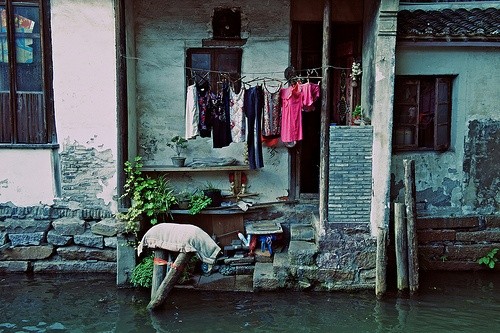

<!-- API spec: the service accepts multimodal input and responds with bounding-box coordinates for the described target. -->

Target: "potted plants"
[166,136,189,168]
[352,105,362,125]
[117,156,222,287]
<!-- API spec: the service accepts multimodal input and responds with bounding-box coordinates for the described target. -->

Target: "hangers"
[185,69,323,83]
